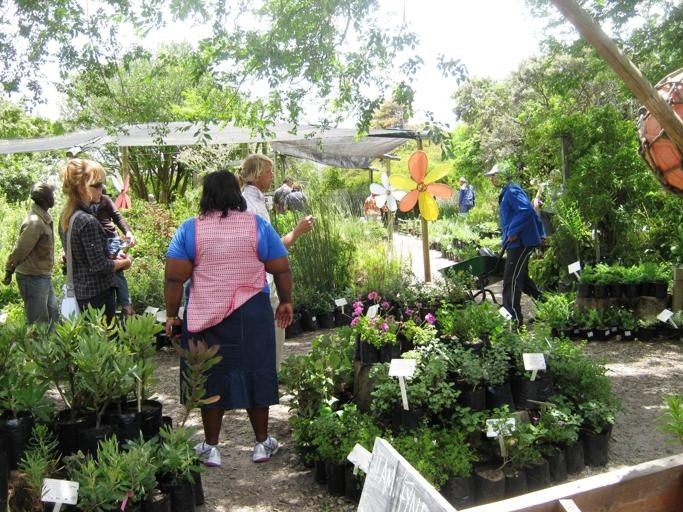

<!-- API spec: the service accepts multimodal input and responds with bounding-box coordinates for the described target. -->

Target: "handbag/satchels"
[61,285,80,317]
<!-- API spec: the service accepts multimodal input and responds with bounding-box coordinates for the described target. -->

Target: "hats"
[484,164,498,176]
[32,183,56,202]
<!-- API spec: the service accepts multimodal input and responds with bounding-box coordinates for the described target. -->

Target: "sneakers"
[253,437,277,460]
[192,442,220,466]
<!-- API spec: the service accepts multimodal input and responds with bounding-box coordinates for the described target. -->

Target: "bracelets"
[166,316,179,320]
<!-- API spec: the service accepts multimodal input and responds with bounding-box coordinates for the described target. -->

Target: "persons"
[234,155,315,373]
[88,196,136,314]
[273,176,294,211]
[459,177,475,213]
[486,165,549,327]
[283,183,309,212]
[534,169,564,238]
[59,159,131,325]
[164,169,293,468]
[363,193,388,218]
[2,186,58,325]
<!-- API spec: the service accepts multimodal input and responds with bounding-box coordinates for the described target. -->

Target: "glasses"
[88,182,102,190]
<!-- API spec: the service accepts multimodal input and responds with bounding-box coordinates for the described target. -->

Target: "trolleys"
[438,236,510,309]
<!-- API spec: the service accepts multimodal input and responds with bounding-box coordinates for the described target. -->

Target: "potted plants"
[277,261,683,511]
[0,300,224,511]
